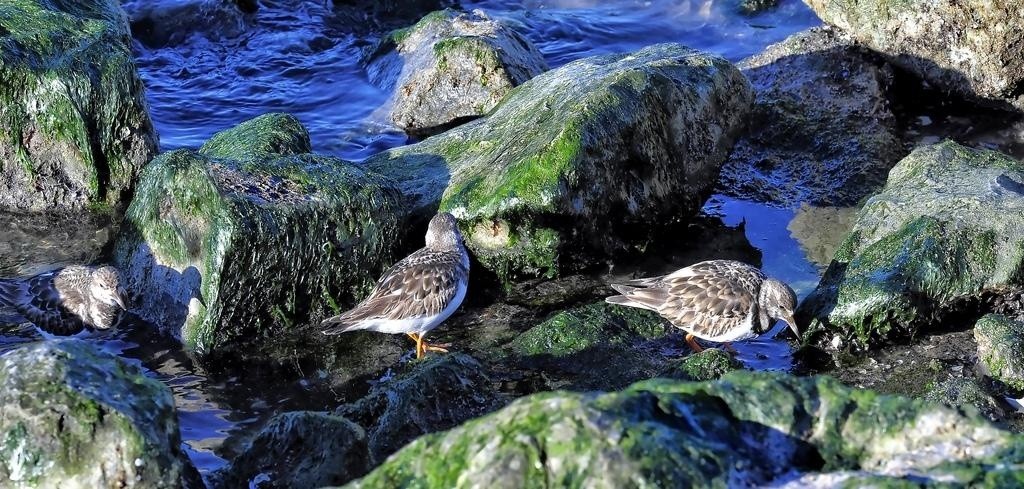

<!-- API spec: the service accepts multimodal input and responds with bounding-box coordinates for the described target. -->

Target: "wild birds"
[603,258,803,356]
[0,263,130,341]
[317,212,472,360]
